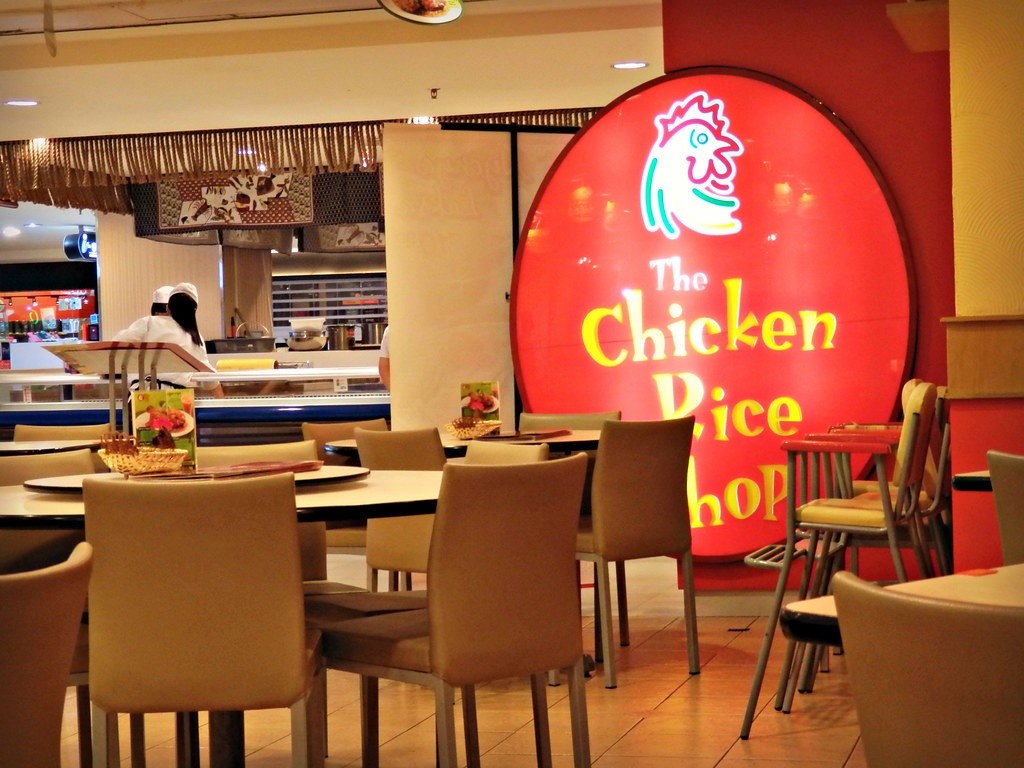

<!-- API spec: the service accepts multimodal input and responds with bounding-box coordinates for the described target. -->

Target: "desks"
[0,439,127,454]
[1,470,441,768]
[325,428,600,457]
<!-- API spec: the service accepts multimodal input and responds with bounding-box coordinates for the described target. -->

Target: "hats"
[152,286,174,303]
[168,282,199,310]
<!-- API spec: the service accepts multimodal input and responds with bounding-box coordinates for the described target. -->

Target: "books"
[127,459,326,481]
[476,430,573,441]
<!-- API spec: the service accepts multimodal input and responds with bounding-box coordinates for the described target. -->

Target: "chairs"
[0,380,1024,768]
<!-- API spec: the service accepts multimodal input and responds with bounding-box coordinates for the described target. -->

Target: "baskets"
[96,432,189,475]
[445,417,502,439]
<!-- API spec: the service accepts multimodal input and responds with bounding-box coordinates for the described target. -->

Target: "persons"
[378,325,389,392]
[112,282,224,443]
[256,380,287,396]
